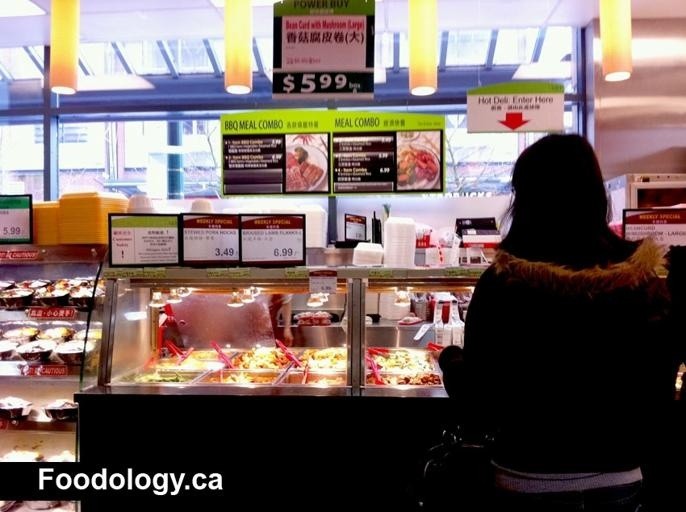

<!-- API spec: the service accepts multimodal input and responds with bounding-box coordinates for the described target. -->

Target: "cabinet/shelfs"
[0,243,151,512]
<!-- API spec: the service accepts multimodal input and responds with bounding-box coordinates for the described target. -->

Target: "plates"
[286,144,330,191]
[398,144,441,191]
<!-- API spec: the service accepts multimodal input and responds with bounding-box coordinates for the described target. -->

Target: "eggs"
[295,311,332,325]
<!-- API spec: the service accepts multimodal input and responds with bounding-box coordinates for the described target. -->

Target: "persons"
[432,131,685,512]
[266,291,297,347]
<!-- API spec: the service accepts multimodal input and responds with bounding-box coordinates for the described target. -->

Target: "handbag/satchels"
[419,443,493,512]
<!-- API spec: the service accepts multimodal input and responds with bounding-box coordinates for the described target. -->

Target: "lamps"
[595,0,634,84]
[143,284,413,317]
[403,0,441,98]
[46,0,82,96]
[222,0,254,98]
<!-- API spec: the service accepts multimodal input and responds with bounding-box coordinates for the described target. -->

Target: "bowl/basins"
[0,275,104,425]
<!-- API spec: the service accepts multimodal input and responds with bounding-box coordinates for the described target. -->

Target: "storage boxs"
[25,197,61,245]
[59,190,126,244]
